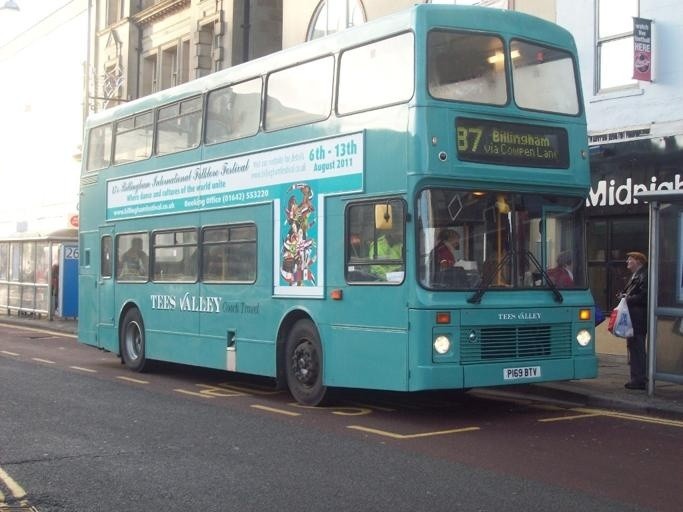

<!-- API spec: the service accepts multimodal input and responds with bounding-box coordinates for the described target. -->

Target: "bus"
[76,3,598,408]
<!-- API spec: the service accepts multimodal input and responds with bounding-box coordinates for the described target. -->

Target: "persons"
[615,250,649,391]
[427,230,460,285]
[369,228,403,284]
[545,251,574,287]
[122,238,149,277]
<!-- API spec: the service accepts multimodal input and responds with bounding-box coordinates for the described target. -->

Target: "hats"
[625,251,647,265]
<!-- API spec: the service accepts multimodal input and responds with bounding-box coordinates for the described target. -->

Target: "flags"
[630,16,652,82]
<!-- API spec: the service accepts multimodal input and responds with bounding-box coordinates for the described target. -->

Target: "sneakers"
[624,381,646,390]
